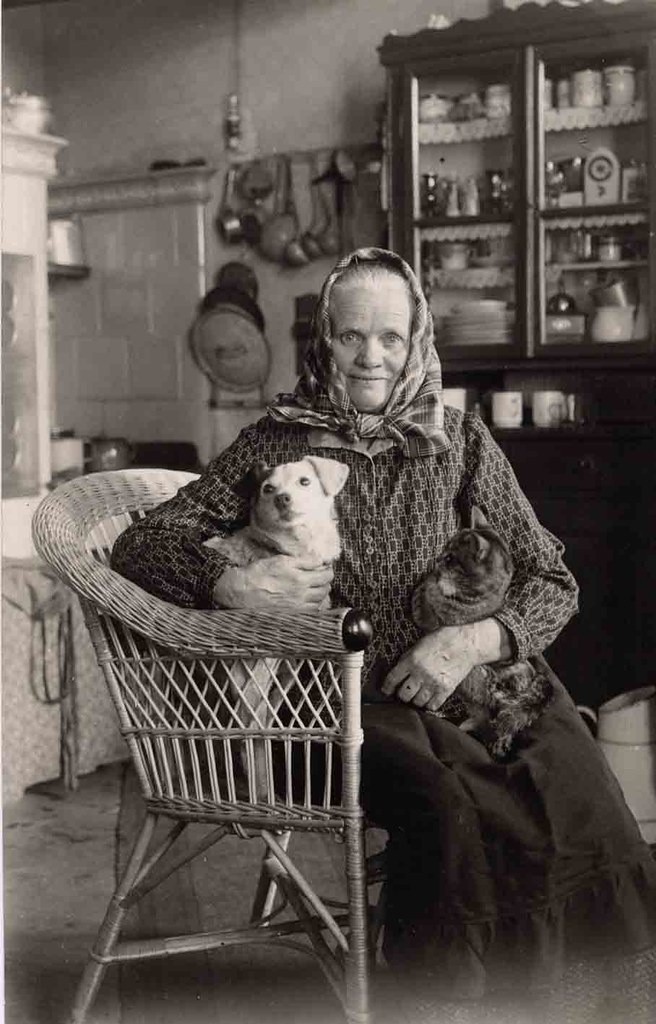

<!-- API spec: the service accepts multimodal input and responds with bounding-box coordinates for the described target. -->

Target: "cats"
[408,505,555,763]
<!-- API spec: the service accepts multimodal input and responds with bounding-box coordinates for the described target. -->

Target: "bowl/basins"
[589,276,639,307]
[590,304,649,343]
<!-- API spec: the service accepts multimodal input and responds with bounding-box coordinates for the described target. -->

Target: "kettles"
[90,435,138,472]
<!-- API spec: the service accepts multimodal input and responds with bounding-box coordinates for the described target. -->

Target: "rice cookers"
[51,429,83,480]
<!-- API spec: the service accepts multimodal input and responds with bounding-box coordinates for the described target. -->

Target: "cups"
[493,391,523,429]
[533,391,575,428]
[419,65,635,124]
[442,388,465,413]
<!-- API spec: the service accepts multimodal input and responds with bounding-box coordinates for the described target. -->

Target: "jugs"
[576,687,656,845]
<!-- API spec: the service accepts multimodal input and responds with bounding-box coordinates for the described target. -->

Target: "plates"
[441,298,515,346]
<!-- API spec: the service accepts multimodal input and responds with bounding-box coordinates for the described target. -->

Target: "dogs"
[201,455,349,804]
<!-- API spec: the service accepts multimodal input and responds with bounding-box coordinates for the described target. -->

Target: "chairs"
[133,439,206,476]
[30,465,395,1024]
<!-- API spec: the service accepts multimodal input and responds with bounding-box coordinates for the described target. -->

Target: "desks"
[0,495,144,801]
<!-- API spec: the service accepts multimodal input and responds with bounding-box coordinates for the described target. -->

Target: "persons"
[110,246,656,986]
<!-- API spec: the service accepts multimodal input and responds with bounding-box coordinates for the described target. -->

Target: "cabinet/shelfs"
[373,0,656,740]
[0,120,70,499]
